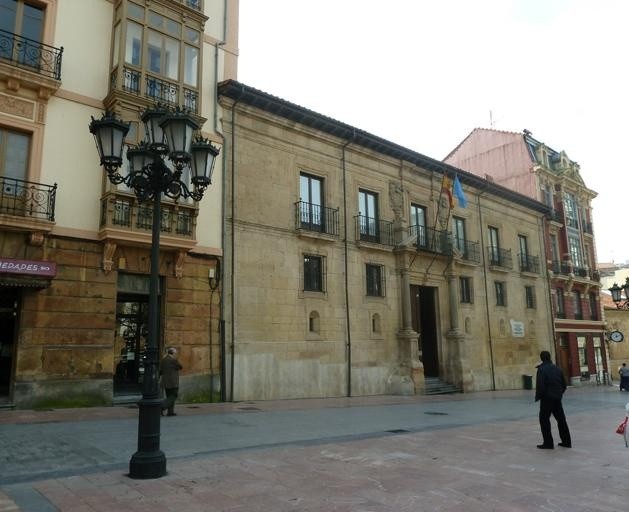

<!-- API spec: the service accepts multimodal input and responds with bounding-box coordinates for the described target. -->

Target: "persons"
[533,350,571,449]
[158,346,183,416]
[618,362,629,392]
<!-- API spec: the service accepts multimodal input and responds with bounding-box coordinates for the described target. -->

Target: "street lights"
[81,96,227,486]
[607,271,629,313]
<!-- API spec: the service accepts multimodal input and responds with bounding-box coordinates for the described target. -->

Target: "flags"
[440,171,455,210]
[452,175,467,210]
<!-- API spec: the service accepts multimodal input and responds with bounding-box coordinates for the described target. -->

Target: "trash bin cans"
[525,376,532,390]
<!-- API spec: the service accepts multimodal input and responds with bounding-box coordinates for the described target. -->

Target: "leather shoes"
[557,443,571,448]
[536,444,553,449]
